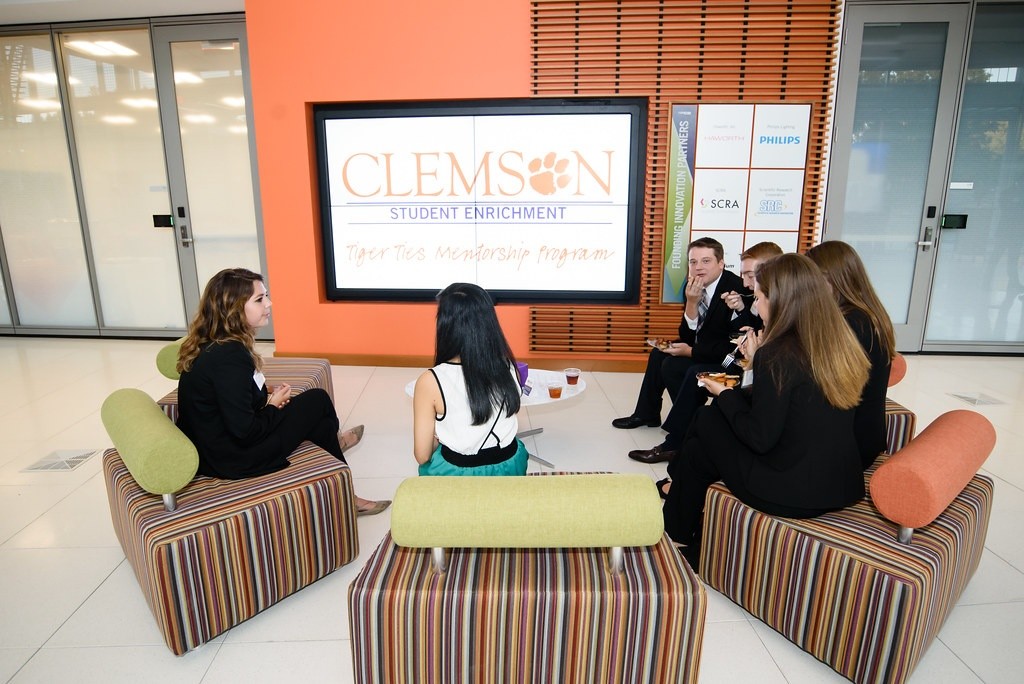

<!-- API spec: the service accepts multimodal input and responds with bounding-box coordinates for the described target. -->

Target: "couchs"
[100,386,358,655]
[346,475,707,684]
[885,350,915,456]
[696,410,996,683]
[155,334,334,426]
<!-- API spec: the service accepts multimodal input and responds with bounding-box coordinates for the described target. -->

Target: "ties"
[696,289,709,331]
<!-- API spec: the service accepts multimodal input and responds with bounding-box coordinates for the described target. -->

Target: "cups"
[548,383,563,398]
[565,368,581,385]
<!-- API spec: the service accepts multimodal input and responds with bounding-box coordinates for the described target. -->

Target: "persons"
[657,252,869,571]
[734,240,895,471]
[612,238,754,430]
[412,283,530,476]
[175,269,392,517]
[628,243,783,464]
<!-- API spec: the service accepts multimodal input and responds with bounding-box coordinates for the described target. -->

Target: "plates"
[728,332,749,340]
[696,371,740,388]
[647,339,669,349]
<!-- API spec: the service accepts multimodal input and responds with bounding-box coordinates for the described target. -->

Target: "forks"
[720,293,753,298]
[722,330,753,367]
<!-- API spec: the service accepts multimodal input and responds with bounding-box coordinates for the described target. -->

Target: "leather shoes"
[613,411,661,429]
[656,480,671,498]
[339,425,364,453]
[354,497,391,516]
[628,443,673,463]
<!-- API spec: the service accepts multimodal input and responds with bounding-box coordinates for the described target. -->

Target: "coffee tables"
[406,369,585,467]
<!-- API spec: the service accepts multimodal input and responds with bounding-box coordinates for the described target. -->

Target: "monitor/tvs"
[314,95,649,306]
